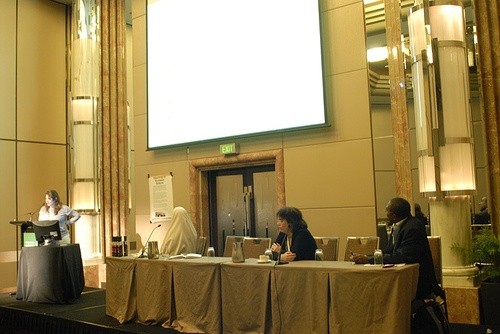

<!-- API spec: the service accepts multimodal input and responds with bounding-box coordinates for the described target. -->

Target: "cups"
[259,254,270,261]
[207,247,215,261]
[374,249,383,268]
[315,249,324,266]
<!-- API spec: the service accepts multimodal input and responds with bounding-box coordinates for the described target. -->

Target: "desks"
[105,256,420,334]
[16,244,85,304]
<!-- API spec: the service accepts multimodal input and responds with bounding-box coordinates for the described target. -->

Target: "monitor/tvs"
[32,220,62,246]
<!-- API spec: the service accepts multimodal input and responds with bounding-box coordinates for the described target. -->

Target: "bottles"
[124,236,128,257]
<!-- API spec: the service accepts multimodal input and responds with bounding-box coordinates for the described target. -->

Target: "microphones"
[276,224,292,265]
[138,224,161,258]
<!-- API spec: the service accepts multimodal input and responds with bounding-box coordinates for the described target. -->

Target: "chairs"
[195,236,449,323]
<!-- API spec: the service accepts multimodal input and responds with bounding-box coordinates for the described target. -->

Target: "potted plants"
[449,226,500,324]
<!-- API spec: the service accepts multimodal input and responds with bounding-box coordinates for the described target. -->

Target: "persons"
[350,197,437,299]
[269,206,321,262]
[38,189,80,243]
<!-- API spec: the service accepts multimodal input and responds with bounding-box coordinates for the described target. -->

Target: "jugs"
[147,242,159,259]
[231,242,245,263]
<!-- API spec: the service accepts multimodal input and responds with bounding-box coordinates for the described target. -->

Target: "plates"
[257,260,273,264]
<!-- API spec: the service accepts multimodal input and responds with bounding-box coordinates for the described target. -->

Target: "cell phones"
[383,264,394,267]
